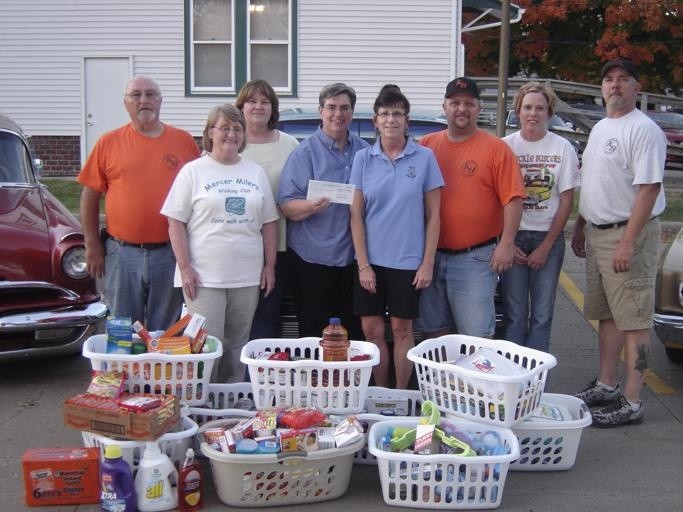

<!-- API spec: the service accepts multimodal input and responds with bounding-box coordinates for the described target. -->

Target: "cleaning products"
[178,447,203,512]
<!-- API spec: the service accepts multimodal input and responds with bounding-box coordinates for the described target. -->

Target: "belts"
[101,233,172,250]
[440,236,503,255]
[588,219,624,229]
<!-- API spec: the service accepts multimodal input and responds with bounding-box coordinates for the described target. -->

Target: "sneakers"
[588,396,642,426]
[575,383,618,404]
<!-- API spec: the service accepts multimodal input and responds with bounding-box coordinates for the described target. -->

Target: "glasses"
[375,111,405,117]
[322,102,353,115]
[212,124,243,132]
[123,91,159,98]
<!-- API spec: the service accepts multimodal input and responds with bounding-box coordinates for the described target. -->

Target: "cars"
[274,108,449,146]
[635,111,683,173]
[502,109,581,160]
[651,226,683,364]
[1,113,109,366]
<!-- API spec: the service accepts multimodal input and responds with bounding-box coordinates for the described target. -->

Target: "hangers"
[388,400,477,457]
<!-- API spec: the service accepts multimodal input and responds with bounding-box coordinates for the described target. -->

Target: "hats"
[443,76,481,99]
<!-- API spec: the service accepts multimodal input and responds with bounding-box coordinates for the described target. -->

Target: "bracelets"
[355,262,370,273]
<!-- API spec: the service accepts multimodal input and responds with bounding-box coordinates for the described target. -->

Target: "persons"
[500,82,581,352]
[350,86,446,389]
[235,79,301,338]
[571,57,667,427]
[417,77,526,340]
[160,105,279,384]
[275,82,372,340]
[77,76,201,330]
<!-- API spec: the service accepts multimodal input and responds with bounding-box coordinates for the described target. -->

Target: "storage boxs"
[81,334,224,405]
[370,417,520,510]
[406,332,556,428]
[442,391,593,474]
[65,393,182,443]
[82,419,201,482]
[183,381,254,459]
[335,387,421,466]
[194,420,367,507]
[22,447,101,508]
[240,336,381,414]
